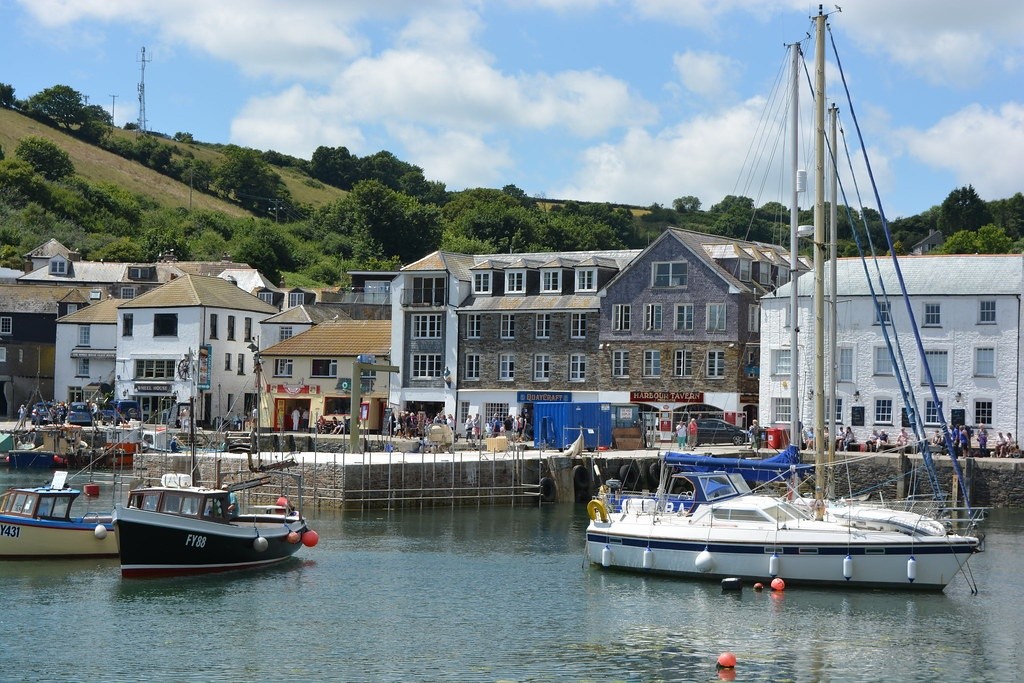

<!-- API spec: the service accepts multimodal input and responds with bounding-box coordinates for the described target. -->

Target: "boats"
[0,423,170,469]
[1,395,204,562]
[110,336,308,579]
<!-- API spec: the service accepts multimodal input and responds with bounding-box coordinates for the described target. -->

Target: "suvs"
[101,399,142,425]
[685,418,751,446]
[31,402,58,424]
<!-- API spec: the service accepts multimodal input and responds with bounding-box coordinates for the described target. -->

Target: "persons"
[931,423,972,458]
[17,405,28,427]
[91,403,99,420]
[689,418,698,451]
[976,421,989,457]
[181,407,190,431]
[676,421,687,449]
[748,419,761,448]
[290,408,309,432]
[233,412,241,429]
[995,433,1016,457]
[488,412,531,443]
[807,425,854,452]
[866,429,889,452]
[316,416,326,433]
[386,411,423,439]
[48,401,68,424]
[465,414,480,442]
[895,428,908,453]
[330,416,347,434]
[251,405,257,431]
[424,411,453,438]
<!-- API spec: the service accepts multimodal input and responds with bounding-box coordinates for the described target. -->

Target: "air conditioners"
[0,316,12,336]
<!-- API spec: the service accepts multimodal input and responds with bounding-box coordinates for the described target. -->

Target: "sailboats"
[588,1,990,592]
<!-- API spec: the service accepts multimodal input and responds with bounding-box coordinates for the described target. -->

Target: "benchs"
[316,421,341,434]
[834,440,995,456]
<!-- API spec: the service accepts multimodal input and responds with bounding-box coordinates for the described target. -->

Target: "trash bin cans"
[767,427,782,449]
[759,428,767,448]
[779,428,790,449]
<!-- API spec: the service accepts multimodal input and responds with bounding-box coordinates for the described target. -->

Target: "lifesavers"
[539,476,556,501]
[587,499,607,522]
[649,462,660,483]
[620,462,640,487]
[572,464,590,489]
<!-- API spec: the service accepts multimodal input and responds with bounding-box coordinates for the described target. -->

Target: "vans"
[68,402,92,425]
